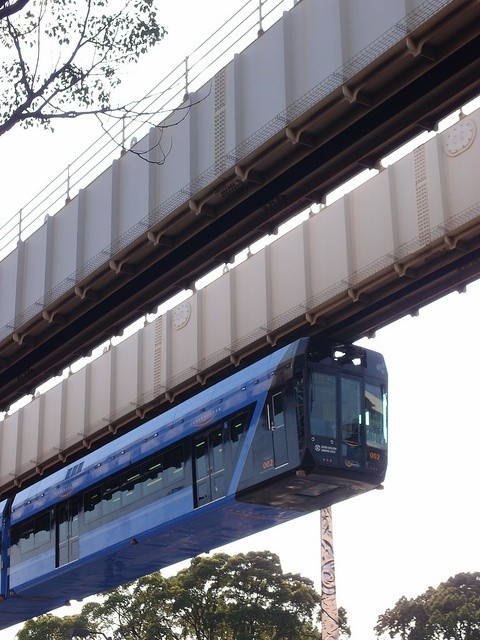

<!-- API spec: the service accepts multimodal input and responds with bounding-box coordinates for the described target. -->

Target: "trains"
[2,336,386,630]
